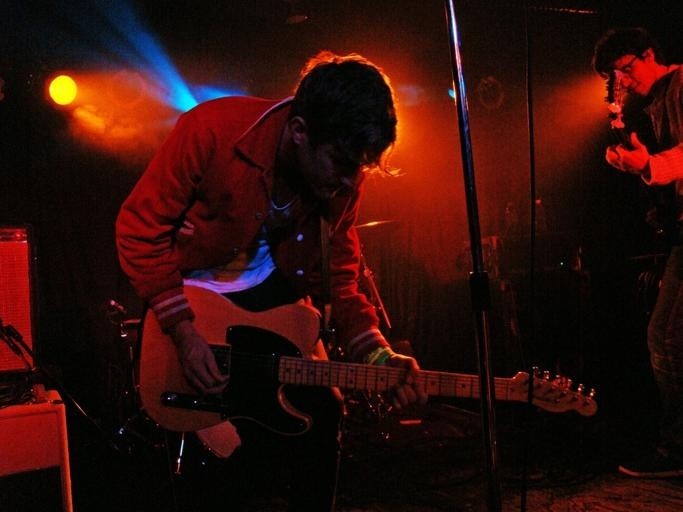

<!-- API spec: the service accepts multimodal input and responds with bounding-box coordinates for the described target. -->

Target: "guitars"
[604,70,675,249]
[132,279,598,436]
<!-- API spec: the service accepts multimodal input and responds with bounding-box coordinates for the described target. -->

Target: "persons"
[591,23,682,479]
[110,52,429,510]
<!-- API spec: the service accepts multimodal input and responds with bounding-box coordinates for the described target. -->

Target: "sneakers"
[615,447,683,479]
